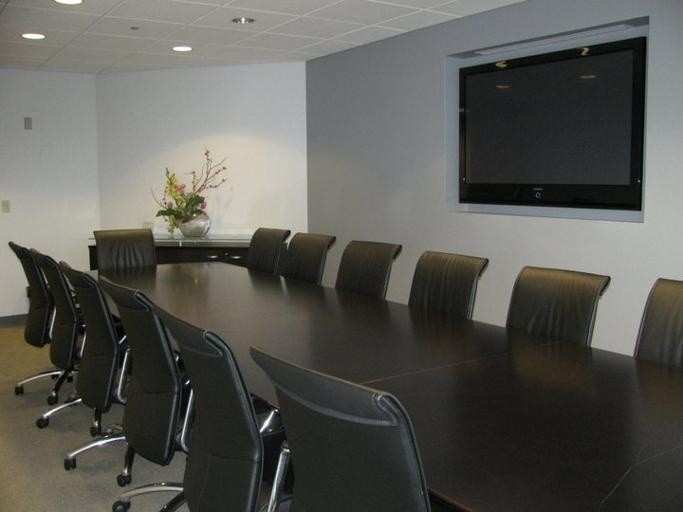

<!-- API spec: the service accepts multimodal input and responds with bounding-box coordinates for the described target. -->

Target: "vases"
[178,208,211,239]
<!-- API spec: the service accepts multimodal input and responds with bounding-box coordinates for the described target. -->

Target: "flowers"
[153,147,228,235]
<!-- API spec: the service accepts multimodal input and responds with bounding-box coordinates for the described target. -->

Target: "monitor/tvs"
[459,35,646,212]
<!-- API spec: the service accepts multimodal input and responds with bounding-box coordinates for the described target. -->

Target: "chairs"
[6,225,682,512]
